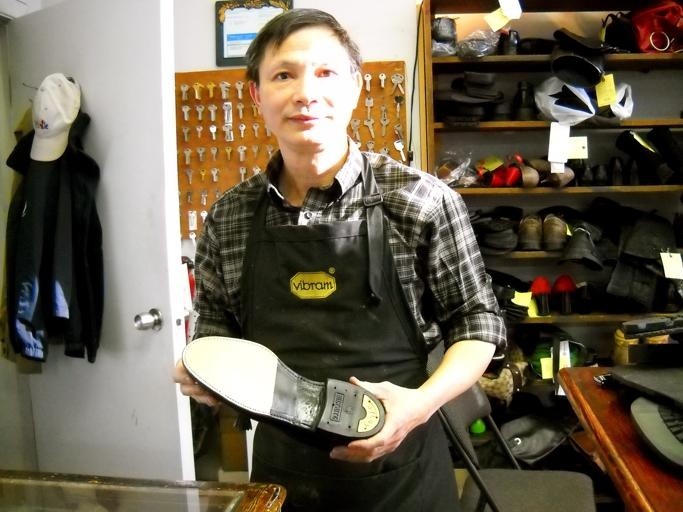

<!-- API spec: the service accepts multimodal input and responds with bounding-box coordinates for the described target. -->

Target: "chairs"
[425,339,599,511]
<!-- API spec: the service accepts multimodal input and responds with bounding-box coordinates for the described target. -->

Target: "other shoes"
[434,29,683,315]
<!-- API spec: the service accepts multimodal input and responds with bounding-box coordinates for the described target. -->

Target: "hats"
[29,72,83,163]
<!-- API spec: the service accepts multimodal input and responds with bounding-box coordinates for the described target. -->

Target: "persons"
[172,8,510,511]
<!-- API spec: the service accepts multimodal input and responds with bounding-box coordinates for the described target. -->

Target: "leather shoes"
[180,334,387,441]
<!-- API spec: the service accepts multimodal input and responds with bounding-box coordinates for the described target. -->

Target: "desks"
[553,364,682,512]
[0,468,290,512]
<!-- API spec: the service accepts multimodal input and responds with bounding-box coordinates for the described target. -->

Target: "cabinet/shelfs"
[416,0,683,329]
